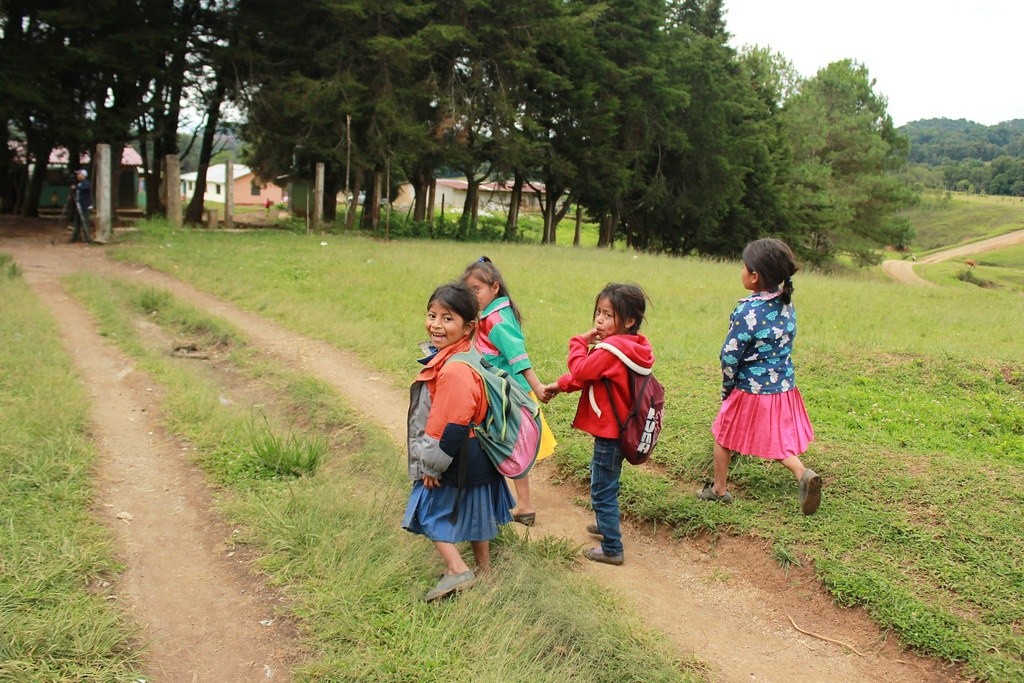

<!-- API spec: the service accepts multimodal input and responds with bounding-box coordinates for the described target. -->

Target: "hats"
[74,169,88,177]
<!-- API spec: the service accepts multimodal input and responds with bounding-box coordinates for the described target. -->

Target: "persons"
[68,169,90,243]
[401,282,517,602]
[458,256,557,528]
[696,238,822,515]
[542,284,655,566]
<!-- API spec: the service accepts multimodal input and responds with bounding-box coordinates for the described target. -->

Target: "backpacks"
[603,365,665,465]
[449,345,543,479]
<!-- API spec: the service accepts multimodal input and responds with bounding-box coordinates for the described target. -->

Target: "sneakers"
[582,547,624,566]
[424,570,476,601]
[695,482,734,505]
[587,524,605,541]
[799,469,822,516]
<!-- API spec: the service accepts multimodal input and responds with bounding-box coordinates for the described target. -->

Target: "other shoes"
[514,512,536,526]
